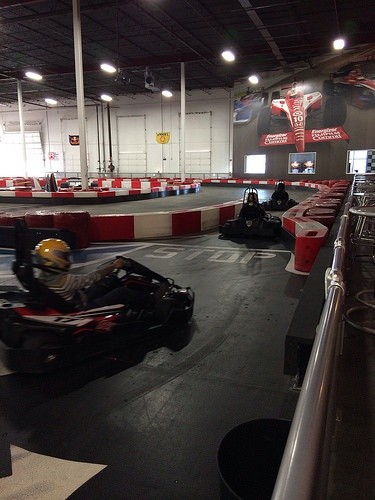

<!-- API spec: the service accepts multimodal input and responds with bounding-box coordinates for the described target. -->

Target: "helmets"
[247,192,258,202]
[276,181,285,191]
[31,238,72,271]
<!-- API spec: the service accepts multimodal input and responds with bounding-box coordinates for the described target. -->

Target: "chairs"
[271,180,289,204]
[11,218,75,313]
[239,188,264,220]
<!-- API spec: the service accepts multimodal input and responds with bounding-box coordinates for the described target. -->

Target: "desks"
[342,178,375,336]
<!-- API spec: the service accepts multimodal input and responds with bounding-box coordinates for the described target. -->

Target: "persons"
[34,238,127,310]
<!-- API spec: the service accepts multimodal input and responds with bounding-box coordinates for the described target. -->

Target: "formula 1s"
[257,82,350,153]
